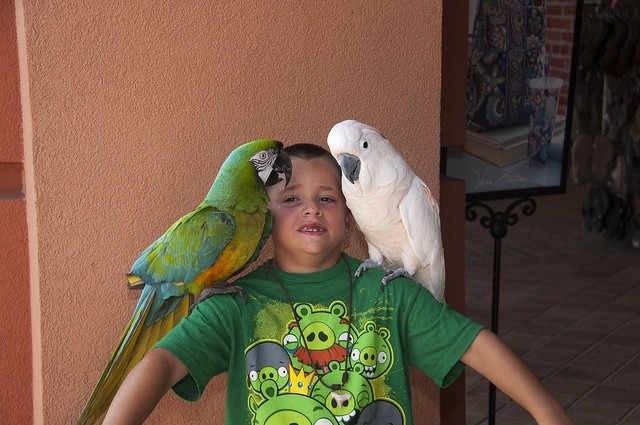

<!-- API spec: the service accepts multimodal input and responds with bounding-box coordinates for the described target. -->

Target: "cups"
[528,76,564,169]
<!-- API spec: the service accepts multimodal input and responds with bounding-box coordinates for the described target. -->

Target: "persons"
[101,143,575,425]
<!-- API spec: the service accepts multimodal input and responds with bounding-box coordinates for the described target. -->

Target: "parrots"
[327,120,447,304]
[76,139,292,425]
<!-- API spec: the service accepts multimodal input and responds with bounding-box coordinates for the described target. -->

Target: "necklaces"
[262,254,353,408]
